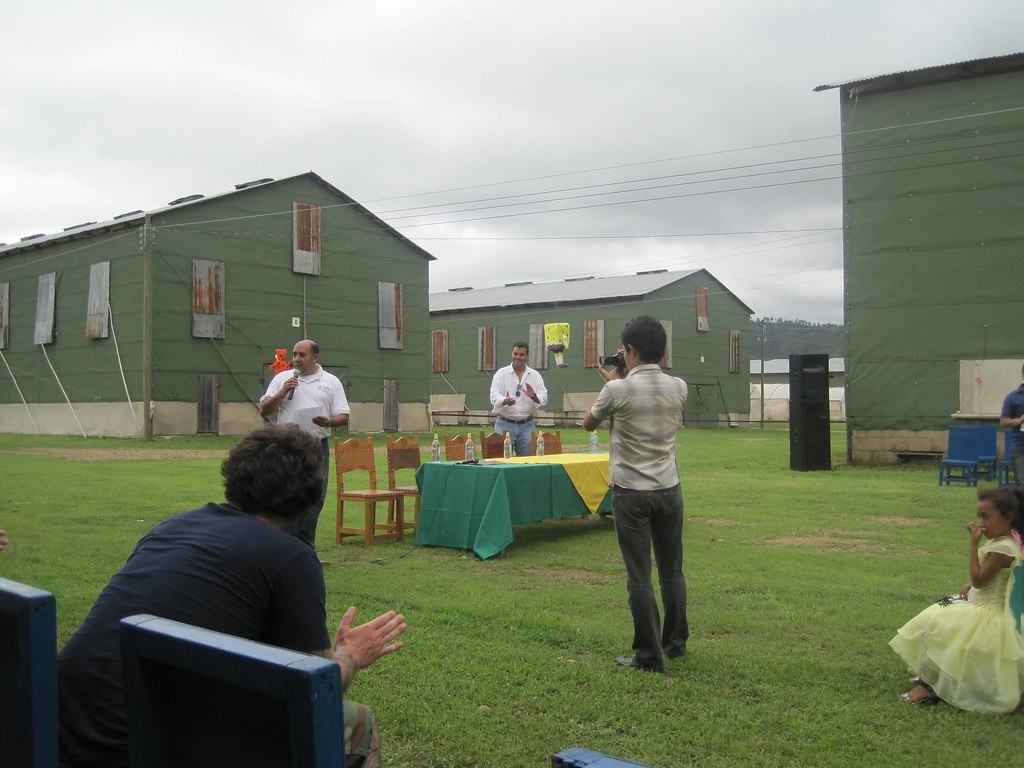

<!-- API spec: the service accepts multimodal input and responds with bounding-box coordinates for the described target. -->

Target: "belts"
[498,413,534,424]
[321,436,327,444]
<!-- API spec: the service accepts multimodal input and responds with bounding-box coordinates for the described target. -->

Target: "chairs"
[532,431,562,455]
[939,426,1017,490]
[0,577,346,768]
[480,430,509,456]
[445,434,475,461]
[334,437,405,547]
[387,435,422,533]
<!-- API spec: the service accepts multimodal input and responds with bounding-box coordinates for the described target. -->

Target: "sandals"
[902,683,947,706]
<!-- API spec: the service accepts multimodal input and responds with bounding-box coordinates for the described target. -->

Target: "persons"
[489,341,548,456]
[56,421,407,768]
[583,314,689,672]
[1000,363,1024,488]
[888,486,1024,715]
[260,340,351,565]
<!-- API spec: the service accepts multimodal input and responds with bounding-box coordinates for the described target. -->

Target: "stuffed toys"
[269,349,289,375]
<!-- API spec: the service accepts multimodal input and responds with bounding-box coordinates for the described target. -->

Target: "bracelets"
[324,418,330,428]
[279,391,286,399]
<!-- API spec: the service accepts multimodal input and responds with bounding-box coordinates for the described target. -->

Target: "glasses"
[512,352,528,357]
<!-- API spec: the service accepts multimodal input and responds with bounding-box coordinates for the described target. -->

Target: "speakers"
[789,353,832,471]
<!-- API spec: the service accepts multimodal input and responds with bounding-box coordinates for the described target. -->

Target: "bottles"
[590,429,599,454]
[432,433,441,463]
[465,433,474,461]
[536,431,545,457]
[503,432,511,459]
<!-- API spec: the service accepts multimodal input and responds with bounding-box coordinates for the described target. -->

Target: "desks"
[413,451,612,560]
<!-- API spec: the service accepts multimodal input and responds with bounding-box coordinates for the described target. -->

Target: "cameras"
[599,351,626,369]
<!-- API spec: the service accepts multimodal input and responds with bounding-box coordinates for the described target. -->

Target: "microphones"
[287,369,300,400]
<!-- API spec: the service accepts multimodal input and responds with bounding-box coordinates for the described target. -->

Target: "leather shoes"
[615,653,657,673]
